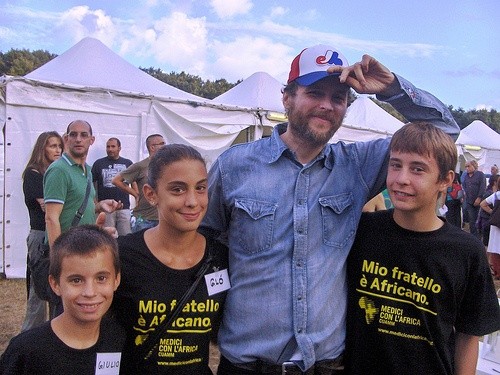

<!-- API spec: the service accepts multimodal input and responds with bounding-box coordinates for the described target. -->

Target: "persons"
[89,137,140,238]
[475,175,494,250]
[112,133,167,232]
[20,130,64,335]
[199,44,460,375]
[489,164,498,176]
[347,120,500,375]
[117,144,229,375]
[481,180,500,296]
[0,224,142,375]
[463,161,486,237]
[43,120,98,325]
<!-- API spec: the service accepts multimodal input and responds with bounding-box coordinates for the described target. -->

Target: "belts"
[220,354,343,374]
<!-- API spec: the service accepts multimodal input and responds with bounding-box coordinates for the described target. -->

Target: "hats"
[287,45,349,86]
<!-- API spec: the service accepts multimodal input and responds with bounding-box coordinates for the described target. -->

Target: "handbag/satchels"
[489,192,500,228]
[27,238,60,305]
[447,183,463,200]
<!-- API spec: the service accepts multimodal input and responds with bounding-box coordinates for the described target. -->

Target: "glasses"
[67,132,91,138]
[151,142,165,145]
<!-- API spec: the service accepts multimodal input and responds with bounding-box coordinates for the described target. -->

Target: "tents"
[1,36,500,279]
